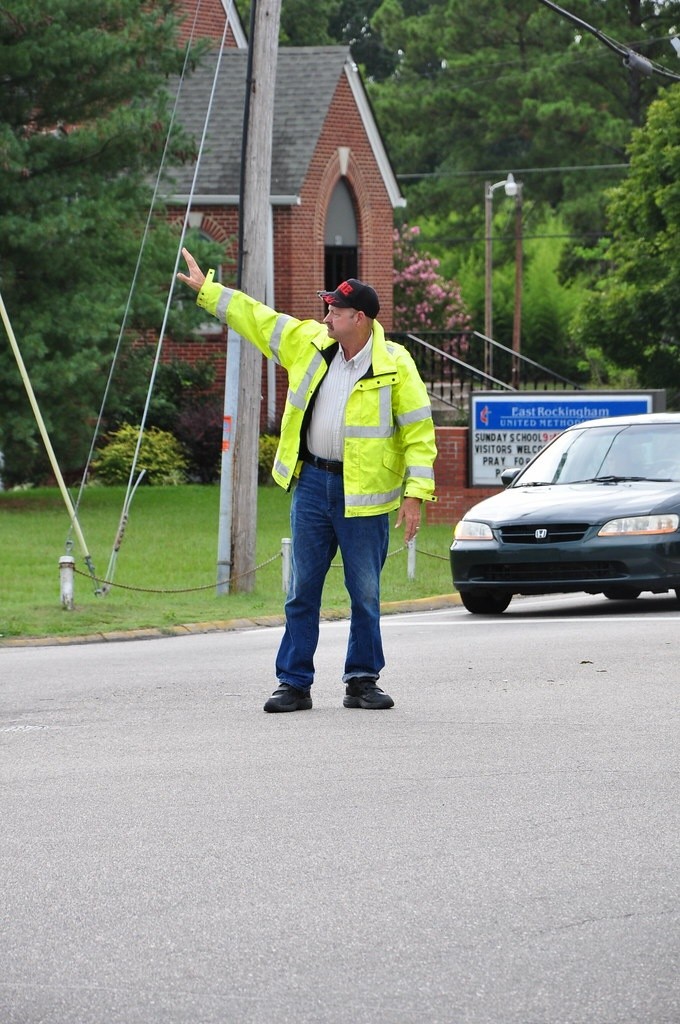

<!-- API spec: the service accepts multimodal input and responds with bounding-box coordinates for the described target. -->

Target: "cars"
[450,412,680,615]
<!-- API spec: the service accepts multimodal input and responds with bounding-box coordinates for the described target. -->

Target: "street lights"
[484,171,520,374]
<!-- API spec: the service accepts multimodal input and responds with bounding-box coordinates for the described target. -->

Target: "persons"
[174,246,439,713]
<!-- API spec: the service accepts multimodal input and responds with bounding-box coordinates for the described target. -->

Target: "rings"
[416,527,420,529]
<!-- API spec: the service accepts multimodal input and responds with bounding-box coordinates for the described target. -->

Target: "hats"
[317,278,380,320]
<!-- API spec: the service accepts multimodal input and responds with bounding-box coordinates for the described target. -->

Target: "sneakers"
[343,677,394,709]
[264,683,313,713]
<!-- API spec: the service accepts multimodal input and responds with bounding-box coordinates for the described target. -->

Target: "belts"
[301,453,343,474]
